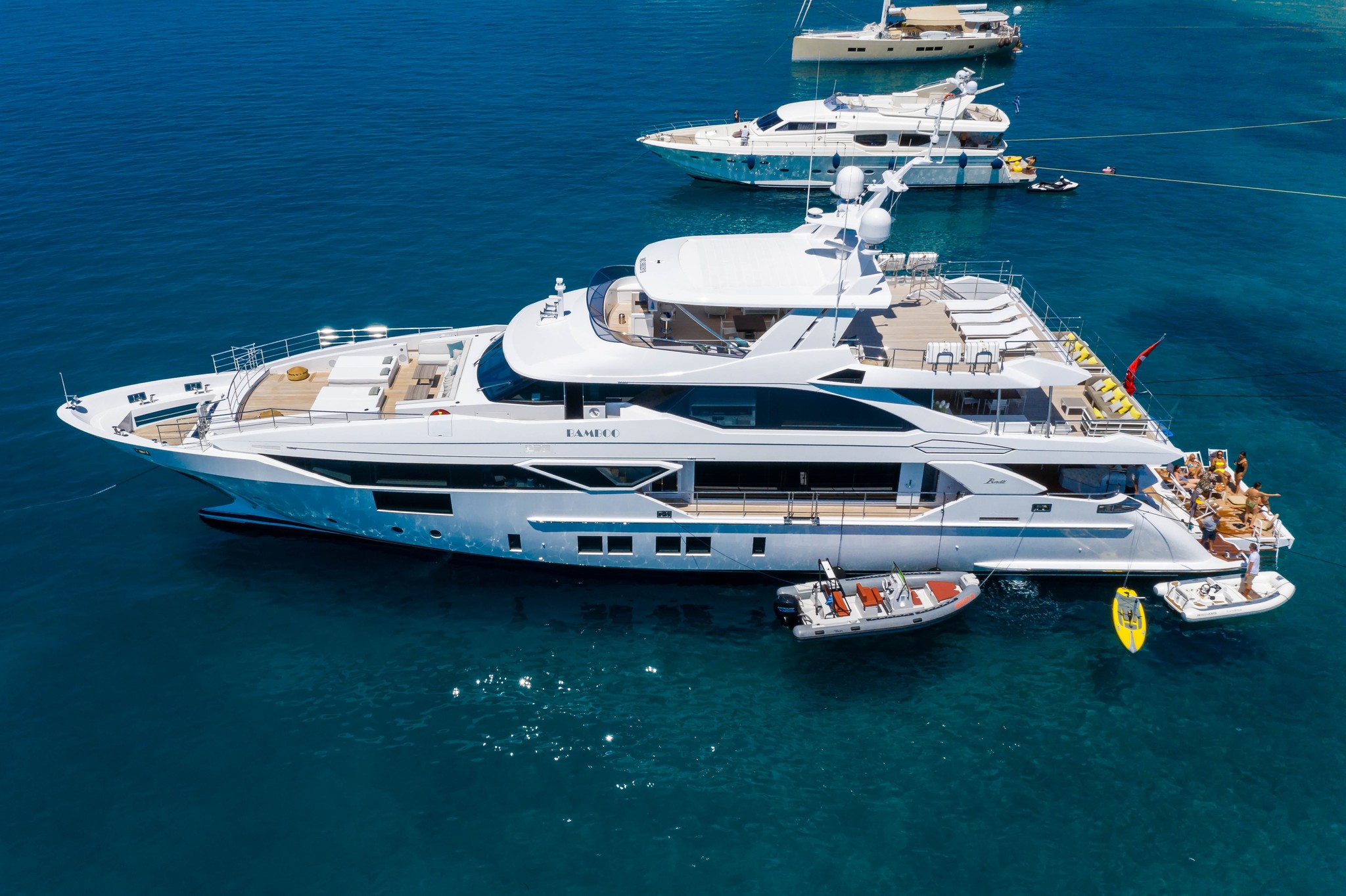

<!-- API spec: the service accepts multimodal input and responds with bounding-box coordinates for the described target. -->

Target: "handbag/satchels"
[1214,484,1226,491]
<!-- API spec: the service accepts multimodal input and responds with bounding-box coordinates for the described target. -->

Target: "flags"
[1122,339,1162,397]
[1011,92,1022,114]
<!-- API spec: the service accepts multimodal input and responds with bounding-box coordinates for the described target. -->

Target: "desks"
[411,364,438,385]
[404,385,432,400]
[1060,397,1086,416]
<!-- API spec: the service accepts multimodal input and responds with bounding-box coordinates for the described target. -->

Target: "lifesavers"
[432,409,449,415]
[944,93,955,101]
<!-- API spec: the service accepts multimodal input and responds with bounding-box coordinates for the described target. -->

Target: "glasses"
[1217,454,1222,456]
[1191,458,1195,459]
[1249,546,1252,548]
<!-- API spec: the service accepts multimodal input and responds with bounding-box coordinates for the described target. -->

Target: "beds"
[309,356,399,421]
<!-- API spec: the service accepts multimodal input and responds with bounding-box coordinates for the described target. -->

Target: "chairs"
[1050,421,1072,436]
[985,30,992,38]
[988,135,993,139]
[940,292,1040,356]
[978,144,987,148]
[703,305,775,344]
[953,389,1027,416]
[1029,421,1052,435]
[1161,447,1279,530]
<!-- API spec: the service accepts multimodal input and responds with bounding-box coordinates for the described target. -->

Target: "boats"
[1152,570,1298,623]
[1027,176,1079,192]
[790,0,1024,63]
[632,66,1038,191]
[764,573,986,645]
[1113,588,1148,654]
[55,77,1296,582]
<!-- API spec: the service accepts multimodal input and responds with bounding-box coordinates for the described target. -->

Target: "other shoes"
[1208,549,1217,554]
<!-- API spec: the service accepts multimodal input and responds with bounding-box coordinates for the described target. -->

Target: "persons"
[1173,464,1200,489]
[740,125,750,146]
[1125,464,1143,499]
[1211,450,1231,490]
[1195,466,1217,496]
[1232,451,1248,496]
[1230,481,1282,529]
[958,132,968,147]
[1111,167,1116,175]
[1199,513,1221,555]
[734,109,741,123]
[1184,453,1203,479]
[1025,156,1037,174]
[1241,543,1260,598]
[1005,157,1011,169]
[1060,176,1067,185]
[1002,20,1025,47]
[992,133,1004,146]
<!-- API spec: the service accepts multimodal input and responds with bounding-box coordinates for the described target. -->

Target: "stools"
[659,308,676,340]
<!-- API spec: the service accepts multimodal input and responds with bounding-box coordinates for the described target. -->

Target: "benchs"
[872,587,883,604]
[396,337,471,404]
[1052,331,1105,370]
[1083,374,1148,435]
[1175,586,1195,601]
[856,583,877,609]
[910,588,922,605]
[1206,577,1248,603]
[824,584,850,616]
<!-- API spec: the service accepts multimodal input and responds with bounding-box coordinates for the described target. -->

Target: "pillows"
[1091,377,1142,421]
[368,340,463,397]
[1058,333,1098,366]
